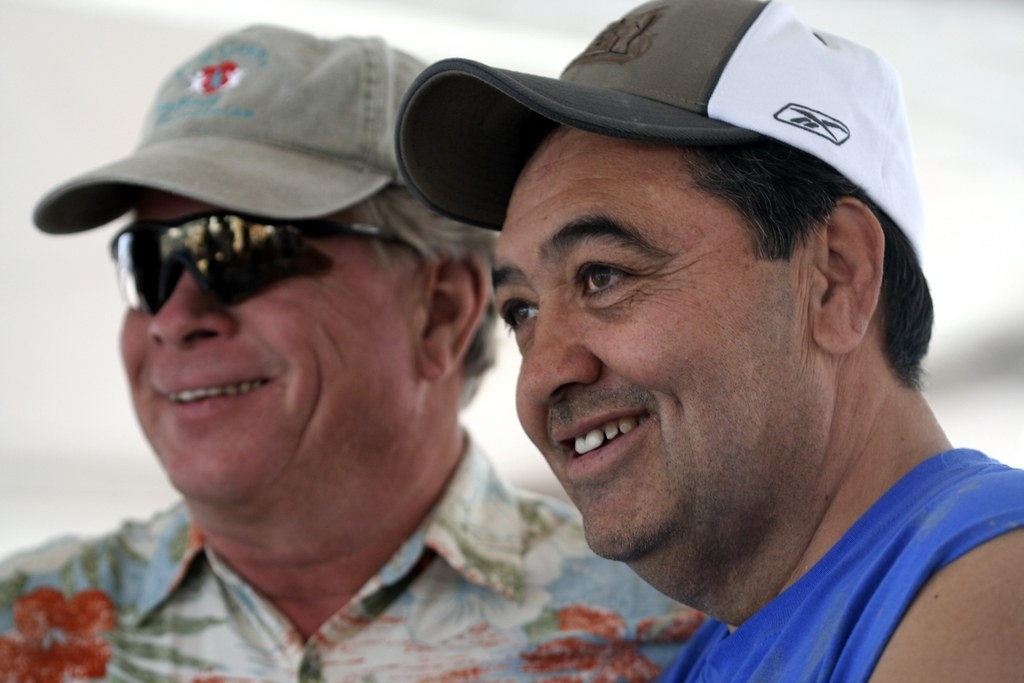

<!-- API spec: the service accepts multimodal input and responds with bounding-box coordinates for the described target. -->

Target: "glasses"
[106,209,410,312]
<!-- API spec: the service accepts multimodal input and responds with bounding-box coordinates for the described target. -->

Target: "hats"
[30,20,429,237]
[392,0,934,334]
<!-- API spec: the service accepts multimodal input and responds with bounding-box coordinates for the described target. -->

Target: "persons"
[392,0,1024,683]
[0,23,705,683]
